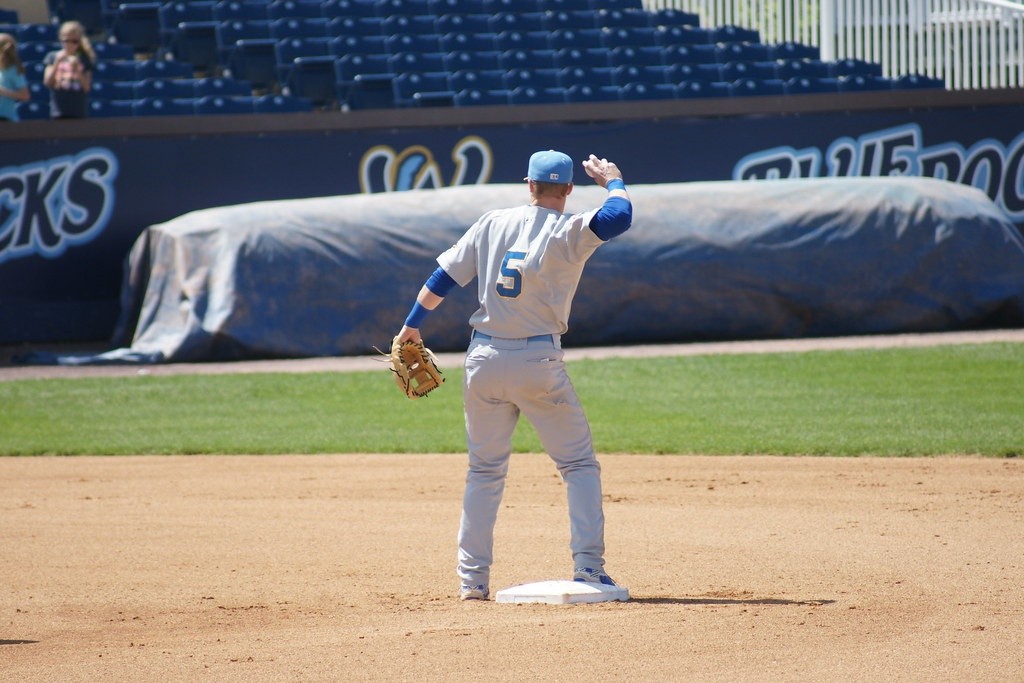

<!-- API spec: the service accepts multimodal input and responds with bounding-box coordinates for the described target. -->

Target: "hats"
[523,150,573,185]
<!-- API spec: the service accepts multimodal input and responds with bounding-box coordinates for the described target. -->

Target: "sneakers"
[573,566,616,585]
[459,584,488,599]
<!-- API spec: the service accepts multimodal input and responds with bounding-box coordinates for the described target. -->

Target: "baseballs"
[583,158,601,178]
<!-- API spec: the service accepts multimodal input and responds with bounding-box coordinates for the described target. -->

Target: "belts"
[473,331,553,342]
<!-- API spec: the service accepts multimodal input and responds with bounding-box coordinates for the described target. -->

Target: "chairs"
[0,0,945,121]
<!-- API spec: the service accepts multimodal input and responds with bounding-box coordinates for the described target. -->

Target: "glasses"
[61,39,80,44]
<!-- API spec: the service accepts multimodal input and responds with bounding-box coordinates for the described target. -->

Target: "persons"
[392,150,633,600]
[44,21,96,118]
[0,33,30,122]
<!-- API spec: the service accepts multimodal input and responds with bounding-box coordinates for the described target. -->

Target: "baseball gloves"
[388,334,446,400]
